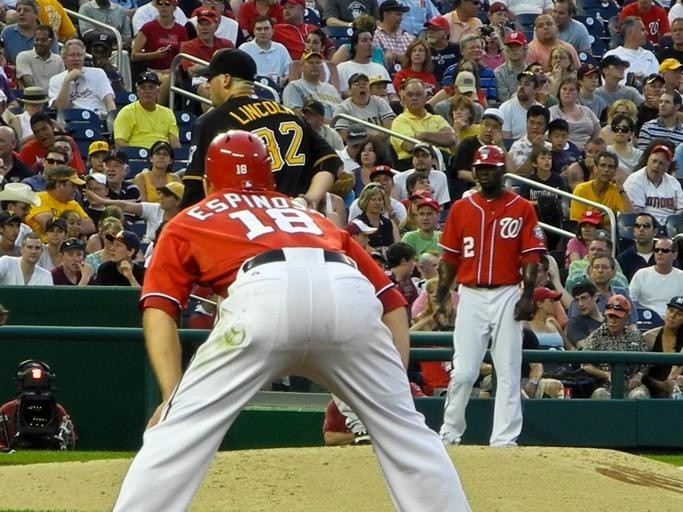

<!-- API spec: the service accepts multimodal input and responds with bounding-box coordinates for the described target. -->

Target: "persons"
[110,130,470,512]
[323,400,354,445]
[1,1,683,398]
[3,359,76,451]
[433,145,547,448]
[178,48,369,444]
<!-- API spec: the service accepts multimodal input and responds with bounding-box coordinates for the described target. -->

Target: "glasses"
[157,2,172,7]
[604,303,628,312]
[633,221,653,228]
[197,13,216,19]
[652,246,674,257]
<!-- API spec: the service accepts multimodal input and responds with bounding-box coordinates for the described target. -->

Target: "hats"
[368,164,395,178]
[570,275,600,304]
[190,46,259,84]
[603,293,631,318]
[195,6,220,24]
[650,143,673,164]
[275,1,682,130]
[416,196,441,213]
[412,142,435,159]
[407,189,433,200]
[577,210,604,228]
[532,285,562,304]
[1,31,187,253]
[665,296,683,312]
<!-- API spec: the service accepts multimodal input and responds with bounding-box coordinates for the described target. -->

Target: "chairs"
[116,146,151,180]
[67,125,104,159]
[519,14,538,41]
[590,1,616,17]
[173,111,198,145]
[578,50,598,65]
[114,91,138,104]
[64,108,101,127]
[575,15,603,35]
[171,148,196,186]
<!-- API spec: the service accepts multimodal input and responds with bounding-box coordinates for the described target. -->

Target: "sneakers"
[353,429,370,444]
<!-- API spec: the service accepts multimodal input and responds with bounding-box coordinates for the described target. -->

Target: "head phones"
[15,359,55,393]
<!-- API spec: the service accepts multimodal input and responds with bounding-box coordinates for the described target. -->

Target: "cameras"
[481,26,493,37]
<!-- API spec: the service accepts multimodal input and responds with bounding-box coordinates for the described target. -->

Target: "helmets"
[202,127,276,194]
[470,143,508,168]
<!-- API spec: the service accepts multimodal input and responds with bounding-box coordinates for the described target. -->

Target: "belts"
[241,245,356,273]
